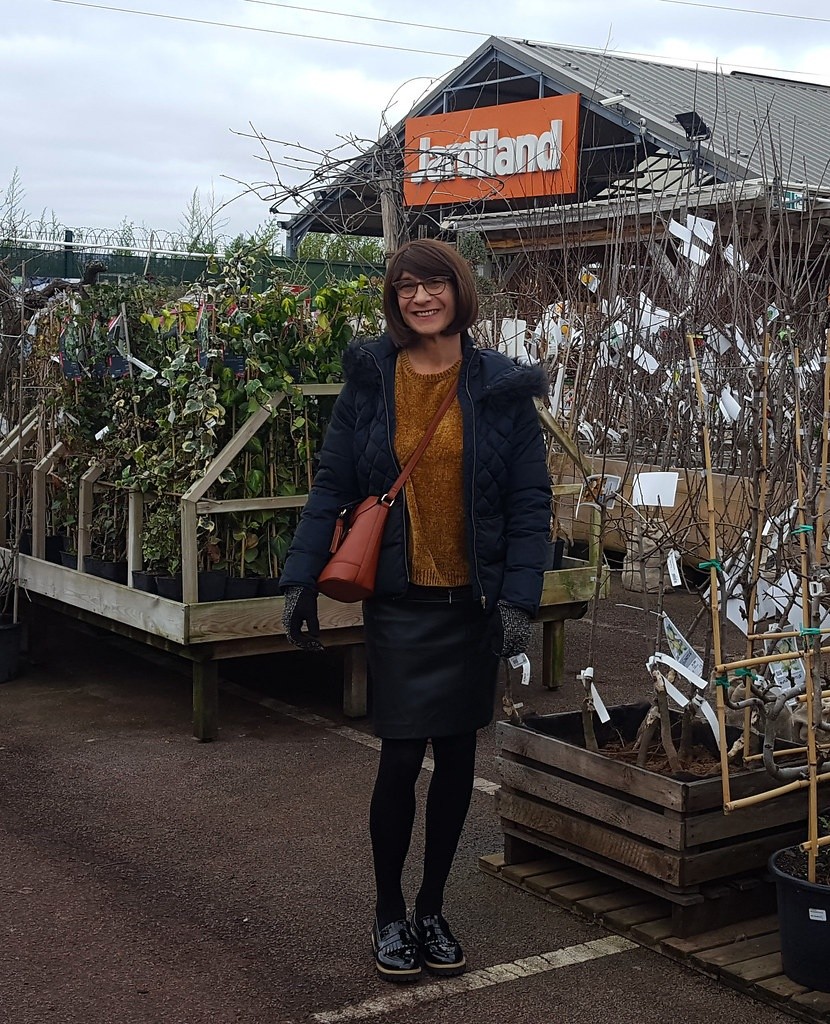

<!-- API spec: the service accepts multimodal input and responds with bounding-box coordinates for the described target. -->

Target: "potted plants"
[17,245,387,604]
[688,325,830,994]
[495,598,830,938]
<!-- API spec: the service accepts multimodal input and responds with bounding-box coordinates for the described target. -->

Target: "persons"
[275,238,566,985]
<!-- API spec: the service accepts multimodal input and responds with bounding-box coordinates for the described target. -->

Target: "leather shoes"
[407,909,466,978]
[368,911,421,985]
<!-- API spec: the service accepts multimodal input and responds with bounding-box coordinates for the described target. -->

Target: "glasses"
[391,276,452,299]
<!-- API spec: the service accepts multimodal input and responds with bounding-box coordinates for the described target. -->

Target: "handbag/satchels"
[317,495,389,603]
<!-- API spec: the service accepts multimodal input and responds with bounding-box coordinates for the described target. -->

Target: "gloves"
[486,600,530,658]
[281,587,323,652]
